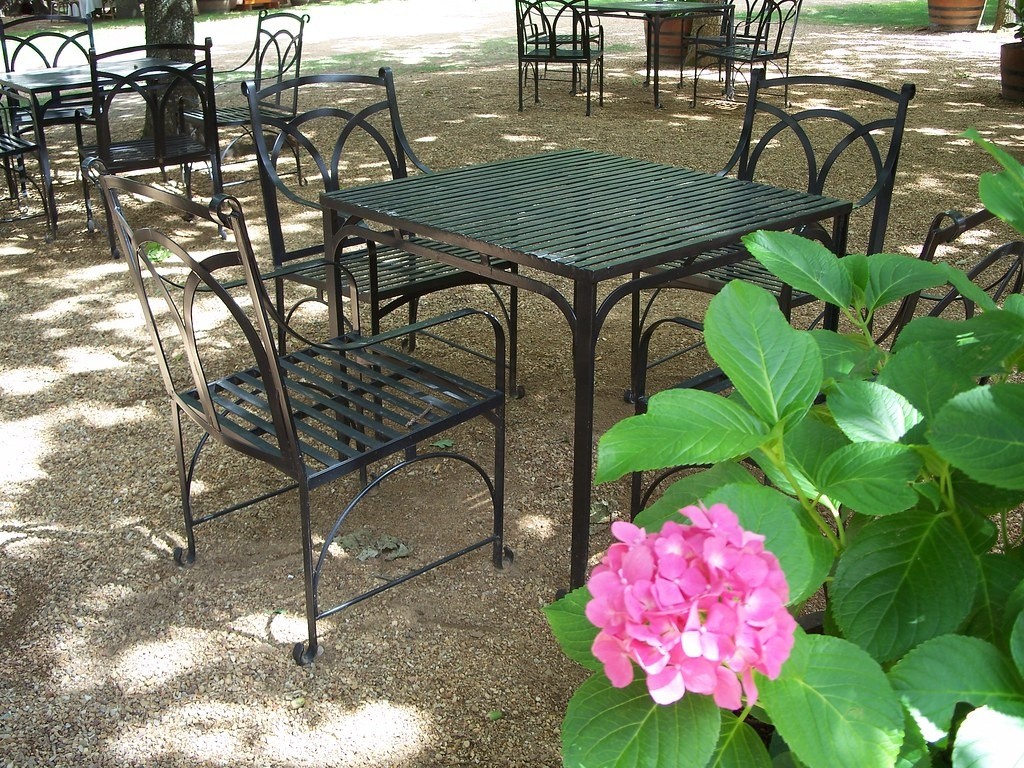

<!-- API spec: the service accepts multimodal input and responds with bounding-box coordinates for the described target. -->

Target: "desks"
[319,148,854,601]
[0,56,199,231]
[569,0,735,109]
[68,0,103,21]
[50,0,81,23]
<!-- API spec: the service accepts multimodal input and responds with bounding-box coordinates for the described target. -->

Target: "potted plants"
[643,0,694,64]
[1000,0,1024,101]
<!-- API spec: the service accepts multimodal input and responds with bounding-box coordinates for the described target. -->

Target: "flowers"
[538,127,1024,768]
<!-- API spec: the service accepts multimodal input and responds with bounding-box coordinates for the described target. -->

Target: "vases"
[928,0,986,33]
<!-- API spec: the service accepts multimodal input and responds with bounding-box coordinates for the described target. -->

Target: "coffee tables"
[101,0,118,20]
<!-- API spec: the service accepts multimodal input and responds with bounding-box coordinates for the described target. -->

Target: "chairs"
[630,209,1024,524]
[0,4,309,260]
[514,0,807,118]
[623,66,916,406]
[79,154,516,668]
[240,64,527,431]
